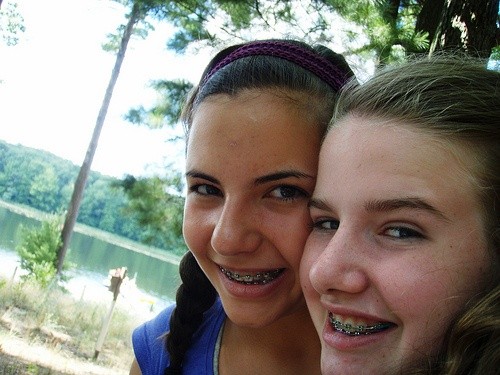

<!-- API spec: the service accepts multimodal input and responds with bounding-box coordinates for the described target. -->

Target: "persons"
[297,47,500,375]
[127,38,361,375]
